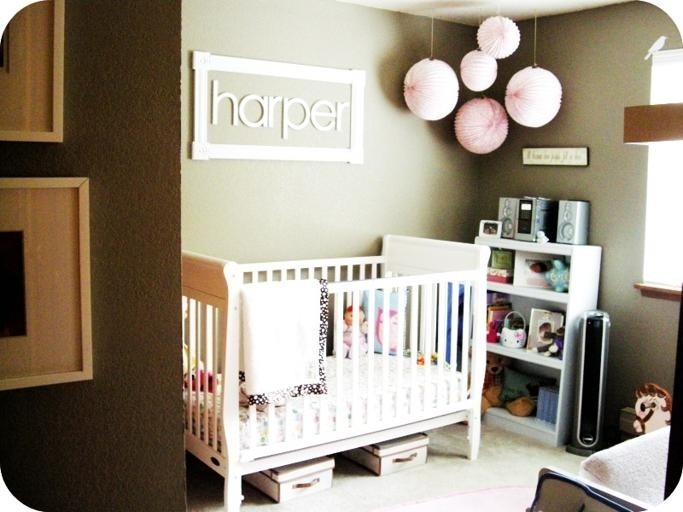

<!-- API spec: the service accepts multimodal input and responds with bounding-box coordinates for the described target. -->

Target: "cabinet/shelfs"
[474,235,602,449]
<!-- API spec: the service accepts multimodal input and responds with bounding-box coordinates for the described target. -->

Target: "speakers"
[556,199,590,245]
[571,309,611,450]
[497,196,518,240]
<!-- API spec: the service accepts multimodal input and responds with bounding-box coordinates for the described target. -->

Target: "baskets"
[500,310,527,348]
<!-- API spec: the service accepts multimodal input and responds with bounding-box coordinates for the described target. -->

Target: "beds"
[181,236,490,512]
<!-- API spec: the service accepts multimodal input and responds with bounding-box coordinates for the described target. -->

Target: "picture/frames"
[0,0,65,143]
[0,178,94,391]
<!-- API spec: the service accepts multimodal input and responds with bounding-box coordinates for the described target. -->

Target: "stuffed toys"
[477,352,535,419]
[333,305,371,360]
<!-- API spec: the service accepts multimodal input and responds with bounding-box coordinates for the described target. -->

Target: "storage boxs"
[242,456,335,504]
[342,431,430,476]
